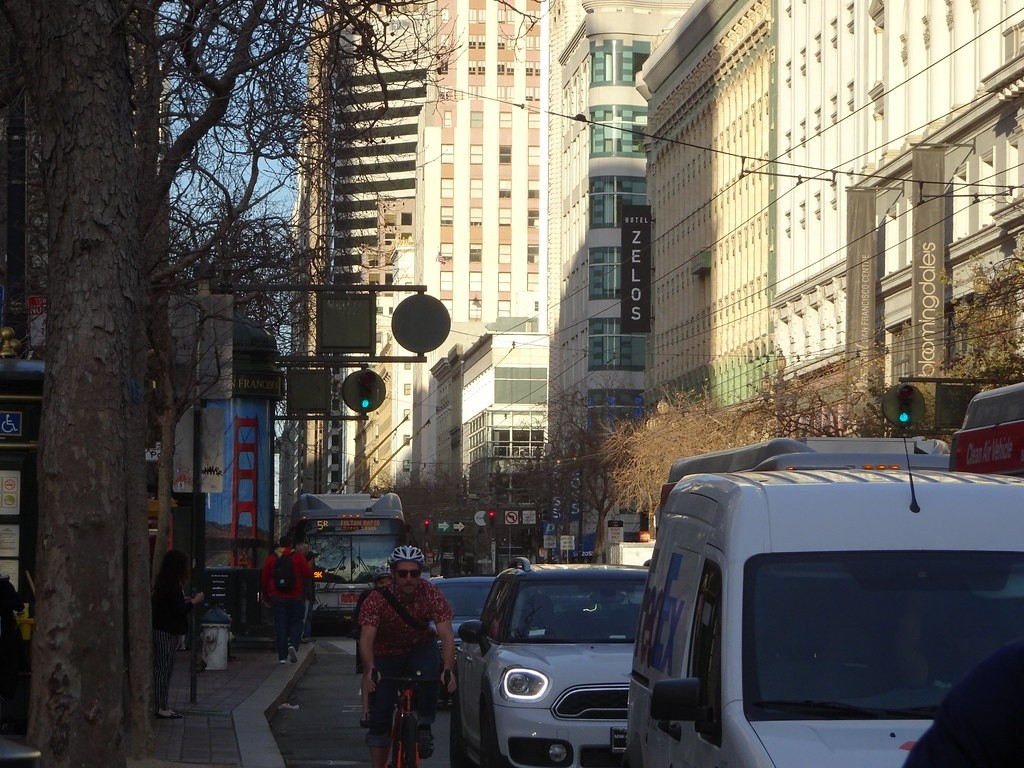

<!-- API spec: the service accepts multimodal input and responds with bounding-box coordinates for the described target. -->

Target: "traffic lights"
[425,520,430,532]
[359,370,374,410]
[489,510,495,526]
[897,383,913,426]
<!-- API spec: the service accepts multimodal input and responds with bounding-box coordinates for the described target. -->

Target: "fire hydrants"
[200,602,234,670]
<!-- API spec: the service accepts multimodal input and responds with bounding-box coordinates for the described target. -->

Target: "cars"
[429,576,498,668]
[450,557,651,768]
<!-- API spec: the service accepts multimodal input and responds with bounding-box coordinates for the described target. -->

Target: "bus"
[287,493,418,637]
[656,380,1024,540]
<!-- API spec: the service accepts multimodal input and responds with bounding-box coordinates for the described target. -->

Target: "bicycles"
[368,668,452,768]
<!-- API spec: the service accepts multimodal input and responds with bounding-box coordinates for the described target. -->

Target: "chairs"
[610,604,640,638]
[514,594,562,639]
[563,603,609,641]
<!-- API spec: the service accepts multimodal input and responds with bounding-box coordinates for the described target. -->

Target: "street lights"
[759,349,786,437]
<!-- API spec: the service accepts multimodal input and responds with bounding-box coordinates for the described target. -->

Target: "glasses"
[395,569,421,578]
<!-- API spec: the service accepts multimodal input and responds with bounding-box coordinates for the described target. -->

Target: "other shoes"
[418,731,434,759]
[279,656,287,664]
[155,711,184,718]
[288,646,298,663]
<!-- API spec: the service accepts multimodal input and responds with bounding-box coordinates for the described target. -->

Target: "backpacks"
[273,551,295,591]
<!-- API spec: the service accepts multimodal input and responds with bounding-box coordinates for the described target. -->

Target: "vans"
[628,469,1024,768]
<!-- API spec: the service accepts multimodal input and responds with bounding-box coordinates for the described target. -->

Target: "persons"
[305,551,319,641]
[0,573,30,734]
[260,536,315,664]
[293,541,310,644]
[357,546,457,768]
[152,548,204,718]
[461,584,492,617]
[354,572,395,728]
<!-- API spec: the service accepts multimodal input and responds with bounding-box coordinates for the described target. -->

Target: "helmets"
[387,545,427,569]
[373,567,392,581]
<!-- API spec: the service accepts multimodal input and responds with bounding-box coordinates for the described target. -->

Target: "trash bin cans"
[204,566,264,633]
